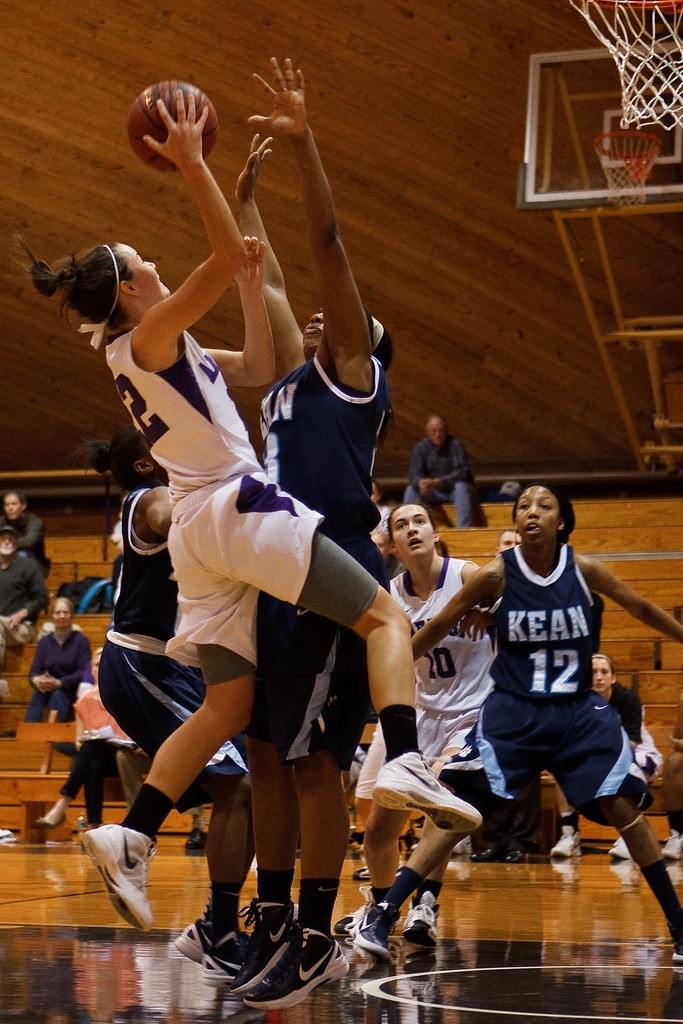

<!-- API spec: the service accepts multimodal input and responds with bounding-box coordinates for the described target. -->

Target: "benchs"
[1,497,683,852]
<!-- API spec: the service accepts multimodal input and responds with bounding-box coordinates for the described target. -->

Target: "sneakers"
[80,822,158,932]
[608,837,631,859]
[660,828,683,860]
[550,825,582,857]
[353,856,402,882]
[244,925,351,1010]
[198,930,250,988]
[369,752,483,834]
[402,892,441,948]
[334,886,376,934]
[175,919,210,964]
[228,898,295,995]
[350,900,402,962]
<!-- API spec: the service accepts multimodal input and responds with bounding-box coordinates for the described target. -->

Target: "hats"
[0,524,20,537]
[498,481,521,497]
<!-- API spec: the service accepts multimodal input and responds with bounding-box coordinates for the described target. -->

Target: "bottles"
[76,812,87,848]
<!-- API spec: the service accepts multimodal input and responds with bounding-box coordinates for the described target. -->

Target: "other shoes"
[30,813,66,830]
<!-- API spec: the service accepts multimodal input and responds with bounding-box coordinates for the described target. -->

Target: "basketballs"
[126,80,219,172]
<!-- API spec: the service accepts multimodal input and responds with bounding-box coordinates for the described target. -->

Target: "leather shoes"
[185,827,206,849]
[471,848,499,861]
[505,850,524,863]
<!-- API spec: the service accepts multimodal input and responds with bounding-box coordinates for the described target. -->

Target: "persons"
[551,653,663,858]
[82,426,256,980]
[117,747,207,849]
[0,491,51,578]
[0,526,50,666]
[28,89,482,930]
[230,58,394,1009]
[353,477,683,960]
[353,718,425,881]
[37,648,134,847]
[335,502,493,946]
[469,772,542,862]
[27,598,95,722]
[369,480,392,560]
[404,415,474,528]
[661,692,683,860]
